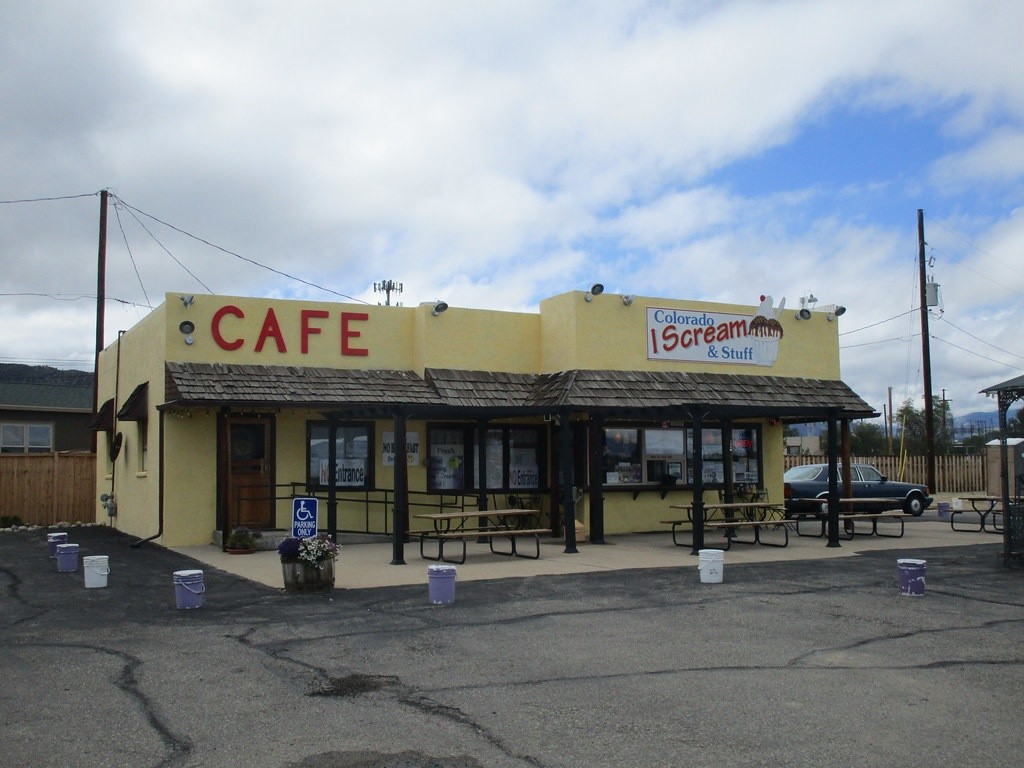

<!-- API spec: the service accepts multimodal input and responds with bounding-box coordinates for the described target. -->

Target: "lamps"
[585,280,604,302]
[180,294,194,306]
[827,304,846,321]
[179,320,195,344]
[619,294,637,305]
[432,298,448,316]
[795,309,812,320]
[804,290,817,307]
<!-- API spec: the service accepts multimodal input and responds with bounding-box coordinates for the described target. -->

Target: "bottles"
[750,484,756,493]
[615,464,641,482]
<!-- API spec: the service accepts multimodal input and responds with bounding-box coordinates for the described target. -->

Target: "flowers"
[274,533,342,571]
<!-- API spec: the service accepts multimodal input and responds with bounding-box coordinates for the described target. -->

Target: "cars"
[783,464,934,517]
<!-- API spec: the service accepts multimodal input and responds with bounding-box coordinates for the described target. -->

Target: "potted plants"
[226,527,258,555]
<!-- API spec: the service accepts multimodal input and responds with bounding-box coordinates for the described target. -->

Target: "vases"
[282,560,334,590]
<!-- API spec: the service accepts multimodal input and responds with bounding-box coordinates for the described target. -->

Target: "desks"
[669,502,789,550]
[421,509,541,564]
[793,496,905,541]
[950,496,1024,535]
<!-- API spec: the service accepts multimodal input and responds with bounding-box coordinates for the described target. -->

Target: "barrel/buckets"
[952,498,962,510]
[897,559,927,597]
[47,533,68,558]
[937,502,949,517]
[987,496,996,509]
[56,543,79,572]
[427,565,456,604]
[173,570,205,609]
[83,555,110,588]
[698,550,724,583]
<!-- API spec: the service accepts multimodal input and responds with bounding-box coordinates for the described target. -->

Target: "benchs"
[660,518,749,524]
[794,511,911,521]
[440,529,552,539]
[707,520,797,527]
[410,525,514,534]
[947,509,1003,516]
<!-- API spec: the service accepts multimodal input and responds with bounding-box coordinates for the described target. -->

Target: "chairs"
[717,489,797,532]
